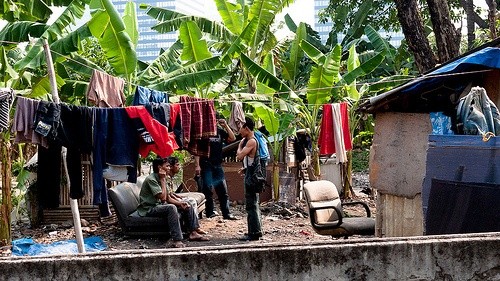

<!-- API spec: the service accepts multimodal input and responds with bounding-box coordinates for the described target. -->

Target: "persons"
[194,113,237,220]
[137,157,210,248]
[237,117,263,241]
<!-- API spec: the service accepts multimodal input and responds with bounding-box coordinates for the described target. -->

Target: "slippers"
[189,234,209,241]
[174,240,185,248]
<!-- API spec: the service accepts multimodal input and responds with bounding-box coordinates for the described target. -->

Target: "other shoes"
[238,232,261,240]
[224,216,241,220]
[208,212,219,219]
[197,229,208,234]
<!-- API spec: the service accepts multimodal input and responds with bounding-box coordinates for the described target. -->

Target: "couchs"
[108,175,207,239]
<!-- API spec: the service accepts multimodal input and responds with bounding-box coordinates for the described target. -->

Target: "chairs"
[303,180,375,240]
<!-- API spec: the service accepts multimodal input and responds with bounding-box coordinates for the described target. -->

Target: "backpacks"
[244,137,267,194]
